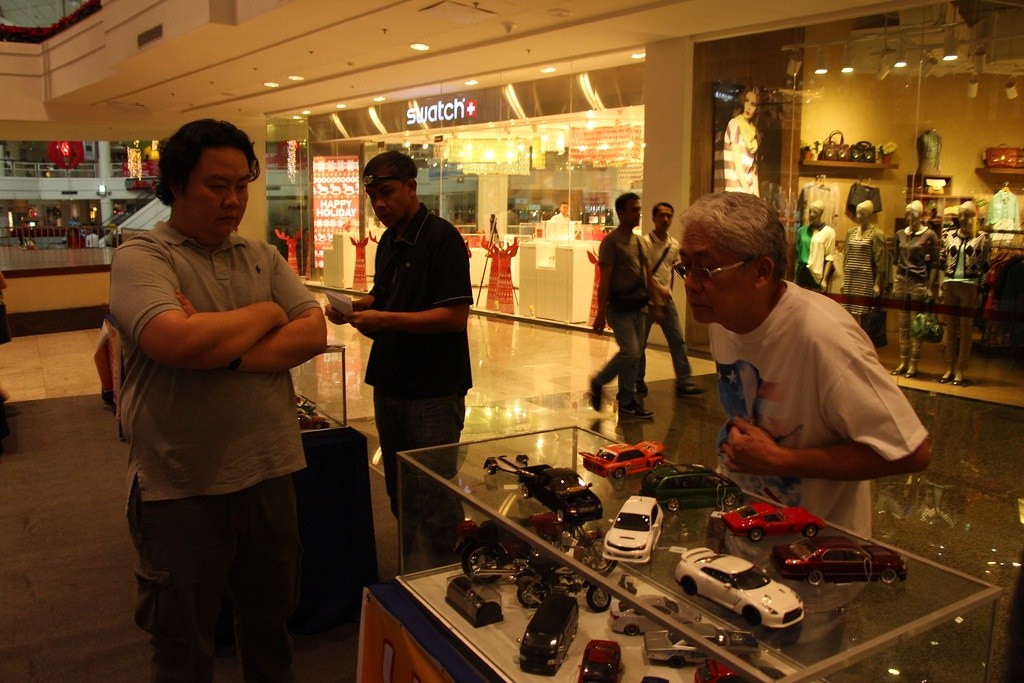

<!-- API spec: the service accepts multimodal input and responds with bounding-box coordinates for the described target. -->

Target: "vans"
[516,593,580,676]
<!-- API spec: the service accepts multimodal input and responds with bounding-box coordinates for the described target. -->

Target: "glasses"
[669,253,760,284]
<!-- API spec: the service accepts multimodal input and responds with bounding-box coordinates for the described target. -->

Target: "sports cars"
[721,502,828,543]
[676,546,806,630]
[577,440,666,479]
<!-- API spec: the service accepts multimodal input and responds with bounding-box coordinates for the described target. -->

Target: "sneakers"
[618,401,656,418]
[589,380,603,412]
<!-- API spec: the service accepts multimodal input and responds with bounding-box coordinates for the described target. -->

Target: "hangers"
[854,175,880,191]
[812,173,831,192]
[987,241,1024,267]
[999,179,1013,195]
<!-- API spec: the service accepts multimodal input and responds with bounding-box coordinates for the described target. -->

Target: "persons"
[67,224,100,248]
[938,201,990,385]
[723,86,761,197]
[325,150,473,576]
[890,200,940,377]
[795,200,836,294]
[672,191,931,667]
[108,118,326,683]
[548,202,575,241]
[637,201,708,401]
[840,200,887,337]
[507,203,519,234]
[0,271,11,405]
[589,193,663,420]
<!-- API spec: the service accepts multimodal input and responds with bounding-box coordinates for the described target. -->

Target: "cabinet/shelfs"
[904,172,977,240]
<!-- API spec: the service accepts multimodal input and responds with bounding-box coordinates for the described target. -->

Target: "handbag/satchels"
[818,132,850,161]
[985,144,1024,167]
[850,141,877,163]
[911,297,944,343]
[858,301,887,347]
[607,282,651,313]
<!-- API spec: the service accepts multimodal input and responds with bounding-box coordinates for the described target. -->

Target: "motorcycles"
[451,511,617,613]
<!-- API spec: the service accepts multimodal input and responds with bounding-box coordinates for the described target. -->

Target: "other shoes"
[615,384,648,399]
[677,383,705,398]
[892,370,907,375]
[906,371,918,378]
[940,375,953,383]
[953,378,964,385]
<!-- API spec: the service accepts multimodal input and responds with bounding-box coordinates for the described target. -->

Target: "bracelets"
[229,357,241,372]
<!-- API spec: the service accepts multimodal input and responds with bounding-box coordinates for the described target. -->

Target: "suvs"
[641,464,744,512]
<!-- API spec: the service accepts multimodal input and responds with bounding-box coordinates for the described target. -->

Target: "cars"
[600,494,665,564]
[772,534,907,585]
[607,593,760,683]
[521,467,604,525]
[579,639,622,683]
